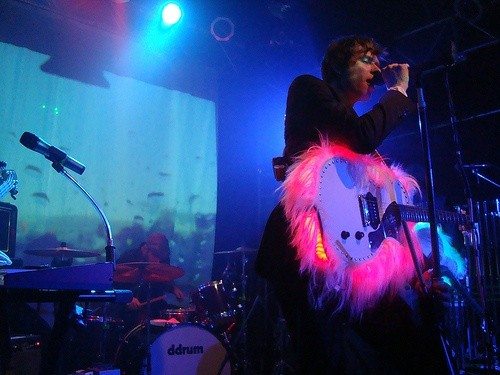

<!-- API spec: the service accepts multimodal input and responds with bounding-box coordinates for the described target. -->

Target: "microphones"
[454,162,490,171]
[19,131,86,175]
[372,57,446,86]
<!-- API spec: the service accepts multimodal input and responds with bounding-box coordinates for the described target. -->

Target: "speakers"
[0,202,18,258]
[4,333,42,375]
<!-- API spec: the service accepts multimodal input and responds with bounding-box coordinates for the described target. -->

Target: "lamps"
[211,18,235,42]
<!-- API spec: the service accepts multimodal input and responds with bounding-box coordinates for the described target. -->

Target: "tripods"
[271,305,296,375]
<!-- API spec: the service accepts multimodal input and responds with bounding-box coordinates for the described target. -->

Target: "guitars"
[283,144,474,298]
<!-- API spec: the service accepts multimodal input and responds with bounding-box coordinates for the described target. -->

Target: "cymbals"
[209,245,259,254]
[23,247,101,258]
[114,261,184,283]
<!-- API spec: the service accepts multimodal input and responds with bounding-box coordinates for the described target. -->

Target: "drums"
[86,311,126,368]
[165,309,195,323]
[114,319,233,375]
[192,280,229,317]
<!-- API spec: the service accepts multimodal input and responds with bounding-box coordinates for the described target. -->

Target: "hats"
[147,233,169,259]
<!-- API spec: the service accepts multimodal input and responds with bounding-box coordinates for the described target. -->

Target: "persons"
[254,37,451,375]
[115,233,184,375]
